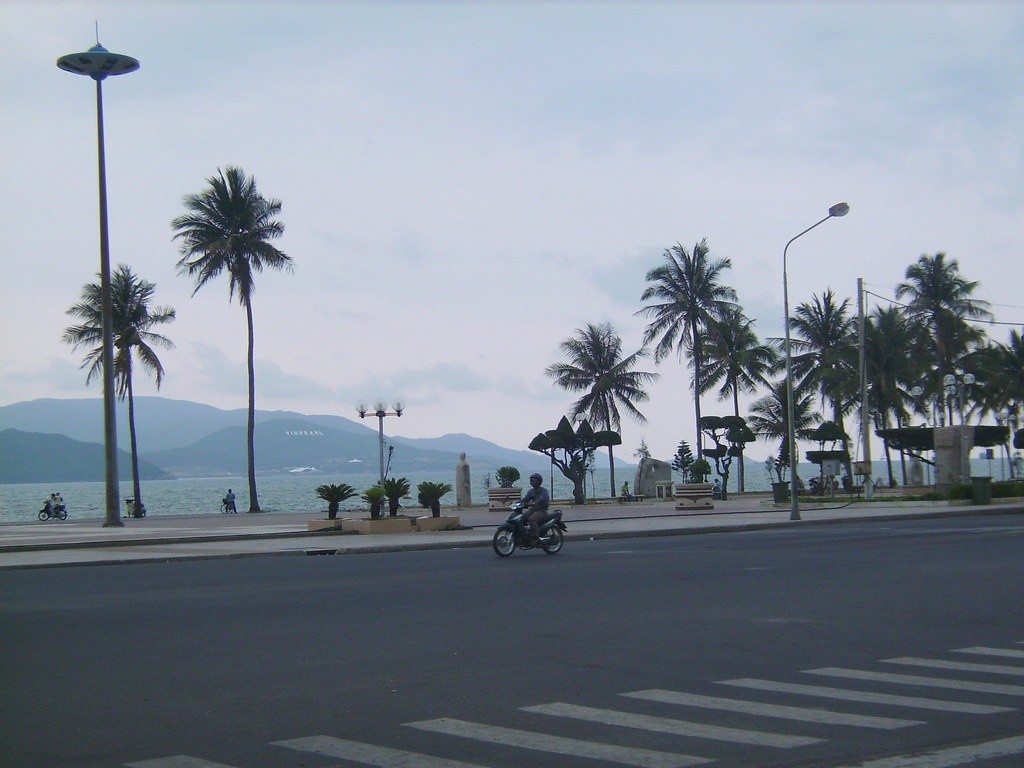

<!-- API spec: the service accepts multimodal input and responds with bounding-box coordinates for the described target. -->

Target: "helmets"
[530,473,542,487]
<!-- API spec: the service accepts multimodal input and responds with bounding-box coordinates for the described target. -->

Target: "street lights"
[355,399,407,517]
[996,412,1015,479]
[783,202,850,520]
[911,388,945,426]
[576,413,588,498]
[943,374,976,423]
[56,42,143,530]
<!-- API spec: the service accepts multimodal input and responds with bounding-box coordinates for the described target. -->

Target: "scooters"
[123,498,147,517]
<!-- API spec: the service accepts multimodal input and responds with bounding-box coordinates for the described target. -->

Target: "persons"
[50,492,62,517]
[621,480,634,503]
[225,489,238,514]
[514,473,550,546]
[714,479,723,492]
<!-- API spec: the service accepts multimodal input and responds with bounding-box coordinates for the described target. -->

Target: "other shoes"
[530,536,540,545]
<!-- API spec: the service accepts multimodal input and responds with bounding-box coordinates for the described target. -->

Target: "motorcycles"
[493,499,568,557]
[37,500,68,520]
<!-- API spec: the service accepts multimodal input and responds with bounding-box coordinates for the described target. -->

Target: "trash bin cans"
[770,481,791,503]
[969,477,993,505]
[655,481,673,502]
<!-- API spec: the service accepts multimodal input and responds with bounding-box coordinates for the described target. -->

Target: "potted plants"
[309,476,460,536]
[485,466,524,513]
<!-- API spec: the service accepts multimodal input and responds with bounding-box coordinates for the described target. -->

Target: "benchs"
[618,495,646,502]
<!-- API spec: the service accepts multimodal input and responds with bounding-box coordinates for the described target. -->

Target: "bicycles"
[220,497,234,514]
